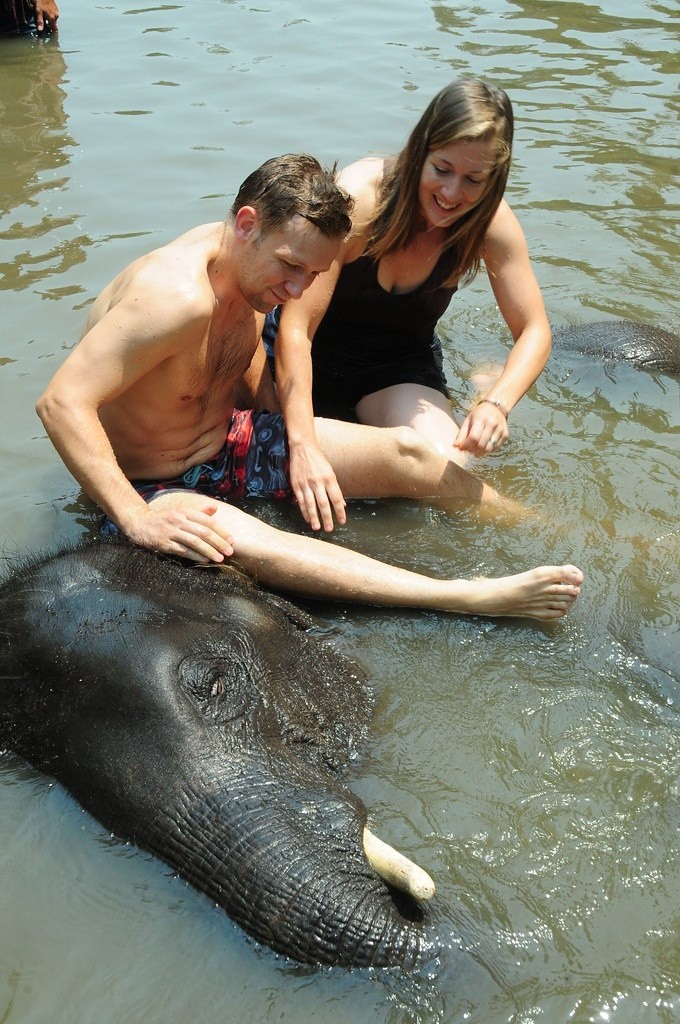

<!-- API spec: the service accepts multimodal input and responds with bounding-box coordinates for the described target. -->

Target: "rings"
[489,437,498,445]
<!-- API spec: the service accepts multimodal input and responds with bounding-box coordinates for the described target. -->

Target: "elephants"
[1,321,680,971]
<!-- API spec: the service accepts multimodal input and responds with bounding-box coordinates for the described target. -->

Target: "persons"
[33,72,590,622]
[0,0,59,40]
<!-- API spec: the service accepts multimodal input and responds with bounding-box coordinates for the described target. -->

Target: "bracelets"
[477,398,509,420]
